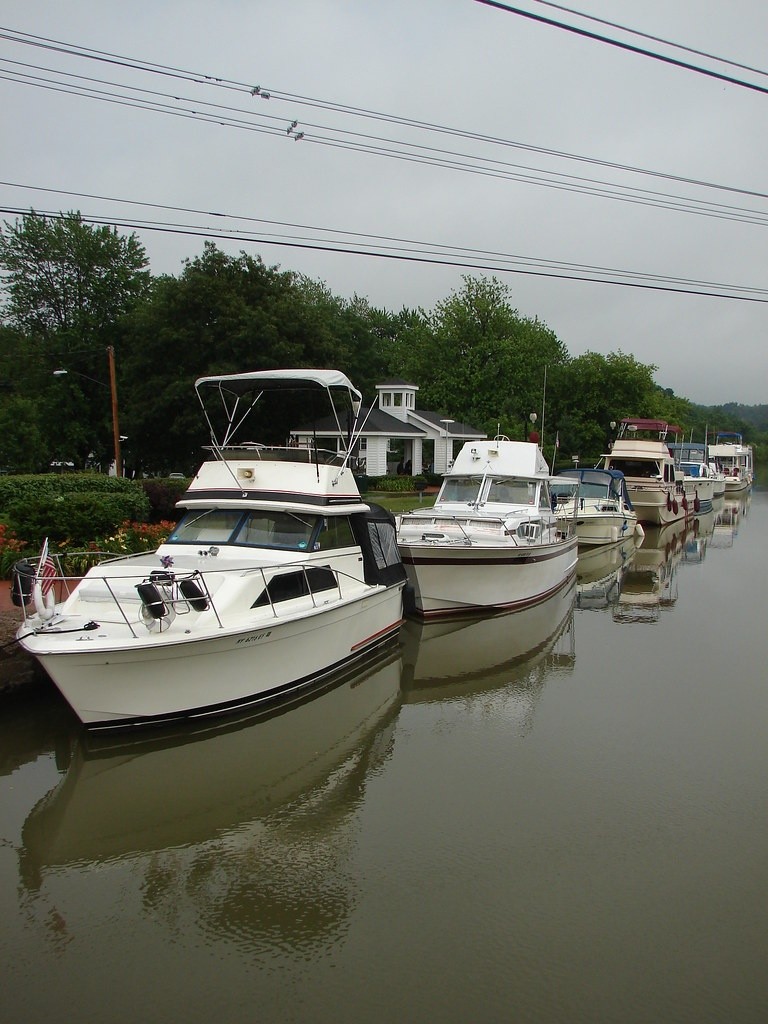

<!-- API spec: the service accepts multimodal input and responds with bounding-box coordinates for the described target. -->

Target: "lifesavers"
[34,579,56,619]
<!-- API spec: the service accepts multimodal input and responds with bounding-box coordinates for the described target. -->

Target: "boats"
[598,417,755,525]
[20,647,409,871]
[577,537,637,584]
[394,423,581,616]
[14,368,408,728]
[550,464,639,547]
[402,573,579,690]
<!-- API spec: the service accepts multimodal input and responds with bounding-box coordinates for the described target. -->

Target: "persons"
[499,480,512,502]
[396,457,413,476]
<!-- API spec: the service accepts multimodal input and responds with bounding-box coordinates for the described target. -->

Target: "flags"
[40,540,57,598]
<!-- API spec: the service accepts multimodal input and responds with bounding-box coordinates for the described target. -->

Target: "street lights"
[53,368,124,476]
[524,407,537,441]
[604,417,617,450]
